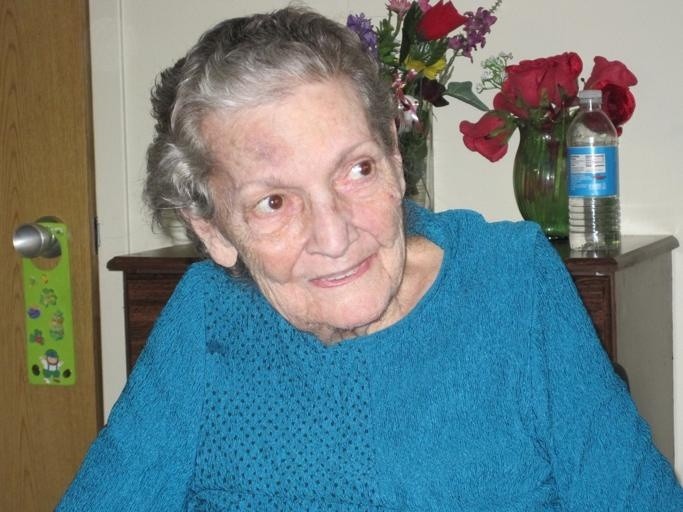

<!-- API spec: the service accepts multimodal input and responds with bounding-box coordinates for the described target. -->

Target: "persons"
[53,5,683,512]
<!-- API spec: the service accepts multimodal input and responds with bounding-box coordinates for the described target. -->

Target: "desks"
[104,235,678,476]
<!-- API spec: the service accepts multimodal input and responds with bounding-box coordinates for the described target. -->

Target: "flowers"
[344,1,504,209]
[459,51,638,163]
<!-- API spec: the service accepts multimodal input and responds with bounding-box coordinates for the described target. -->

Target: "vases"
[511,117,571,238]
[396,105,434,212]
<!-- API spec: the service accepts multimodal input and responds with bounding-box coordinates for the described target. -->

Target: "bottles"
[565,88,622,252]
